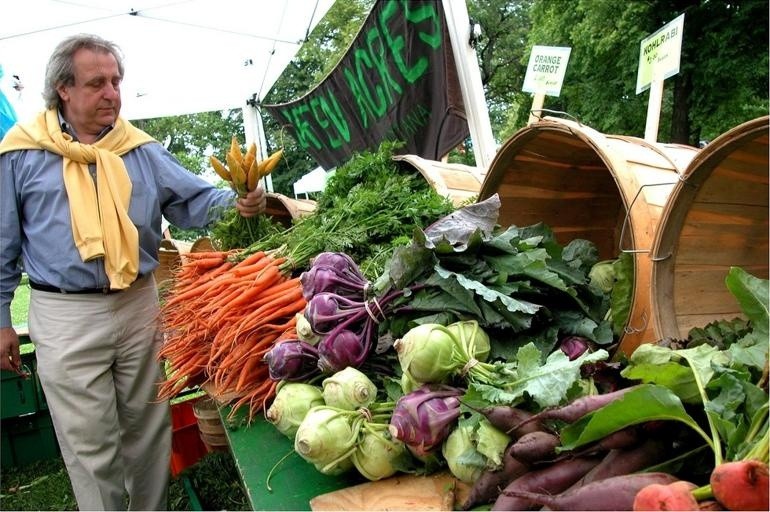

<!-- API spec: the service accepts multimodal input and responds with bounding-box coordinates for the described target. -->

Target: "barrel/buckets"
[389,153,487,213]
[619,116,768,350]
[473,108,702,363]
[260,190,318,226]
[154,238,191,284]
[193,398,227,458]
[189,235,221,255]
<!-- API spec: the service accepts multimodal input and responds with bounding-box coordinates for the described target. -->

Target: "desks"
[170,384,373,511]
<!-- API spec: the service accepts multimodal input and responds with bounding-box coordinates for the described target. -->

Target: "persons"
[1,32,267,511]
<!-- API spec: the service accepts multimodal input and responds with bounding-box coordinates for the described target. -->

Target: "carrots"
[208,137,284,192]
[144,247,310,429]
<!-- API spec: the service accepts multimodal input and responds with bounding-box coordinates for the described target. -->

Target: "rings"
[258,204,262,212]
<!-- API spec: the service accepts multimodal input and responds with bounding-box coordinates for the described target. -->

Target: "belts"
[29,272,144,296]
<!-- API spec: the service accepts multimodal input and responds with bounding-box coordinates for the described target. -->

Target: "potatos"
[456,383,679,511]
